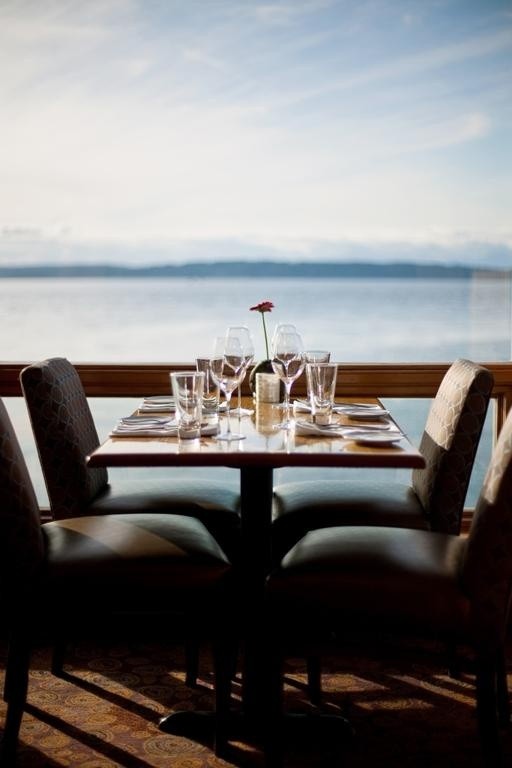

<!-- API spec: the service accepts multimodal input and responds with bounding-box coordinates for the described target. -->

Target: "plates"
[341,424,404,448]
[108,415,178,438]
[333,403,390,421]
[137,395,176,414]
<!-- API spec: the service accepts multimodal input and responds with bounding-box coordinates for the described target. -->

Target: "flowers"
[249,301,275,362]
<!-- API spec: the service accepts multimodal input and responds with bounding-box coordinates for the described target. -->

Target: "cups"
[194,353,226,417]
[255,372,281,404]
[301,349,338,427]
[169,370,206,441]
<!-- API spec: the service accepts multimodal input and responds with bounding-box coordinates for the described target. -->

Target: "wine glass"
[227,325,255,417]
[207,353,246,442]
[268,323,307,432]
